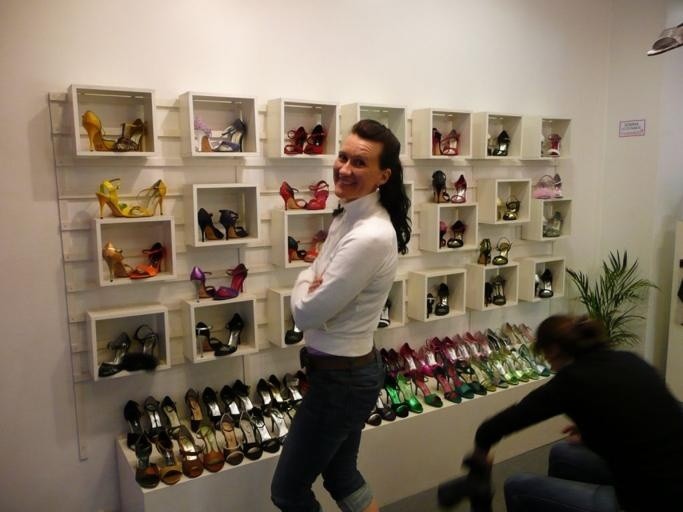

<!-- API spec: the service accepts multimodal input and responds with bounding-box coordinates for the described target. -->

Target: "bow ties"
[332,203,344,217]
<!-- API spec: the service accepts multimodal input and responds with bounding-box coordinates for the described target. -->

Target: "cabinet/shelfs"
[408,109,571,321]
[403,179,414,235]
[376,276,405,332]
[342,103,408,161]
[71,87,342,380]
[115,372,575,511]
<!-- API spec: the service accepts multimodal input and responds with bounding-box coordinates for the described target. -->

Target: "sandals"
[122,372,303,490]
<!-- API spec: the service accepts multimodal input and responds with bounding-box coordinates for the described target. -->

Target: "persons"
[461,314,683,512]
[271,119,411,512]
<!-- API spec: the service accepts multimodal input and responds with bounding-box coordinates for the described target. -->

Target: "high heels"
[433,128,460,155]
[485,276,506,308]
[95,177,167,218]
[288,231,327,263]
[82,110,147,152]
[194,118,246,152]
[103,240,165,282]
[190,263,248,301]
[440,219,464,249]
[432,170,468,204]
[365,322,551,426]
[477,237,512,266]
[195,312,245,358]
[280,181,329,210]
[198,207,249,243]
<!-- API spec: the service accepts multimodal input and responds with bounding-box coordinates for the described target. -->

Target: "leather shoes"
[534,174,562,199]
[378,300,392,328]
[285,124,327,154]
[427,282,449,316]
[99,324,161,377]
[285,319,303,345]
[488,130,510,156]
[503,194,521,220]
[535,268,553,298]
[547,133,561,155]
[543,212,562,237]
